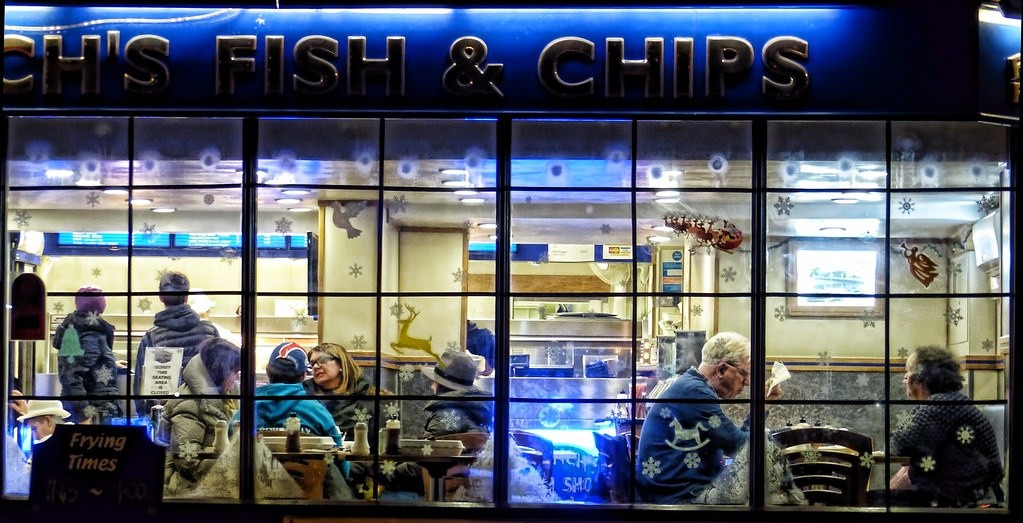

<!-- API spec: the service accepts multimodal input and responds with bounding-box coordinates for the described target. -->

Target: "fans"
[588,261,650,286]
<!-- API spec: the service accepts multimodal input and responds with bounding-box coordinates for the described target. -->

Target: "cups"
[587,299,602,314]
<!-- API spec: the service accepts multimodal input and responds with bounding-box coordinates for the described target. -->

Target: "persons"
[636,330,784,503]
[303,343,389,496]
[891,346,1006,494]
[133,271,218,417]
[53,287,128,421]
[164,336,239,480]
[10,388,75,490]
[228,341,350,503]
[380,350,493,495]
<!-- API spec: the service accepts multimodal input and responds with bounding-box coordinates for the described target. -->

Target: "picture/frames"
[786,237,888,316]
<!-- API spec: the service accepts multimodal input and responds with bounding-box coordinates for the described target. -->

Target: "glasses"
[310,356,335,368]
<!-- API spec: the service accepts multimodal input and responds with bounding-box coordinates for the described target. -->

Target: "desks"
[197,453,327,500]
[343,453,479,501]
[784,449,913,506]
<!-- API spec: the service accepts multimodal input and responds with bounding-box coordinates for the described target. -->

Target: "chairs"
[254,430,555,502]
[591,429,1003,507]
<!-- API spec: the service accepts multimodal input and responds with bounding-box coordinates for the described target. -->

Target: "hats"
[269,342,308,373]
[16,399,71,423]
[421,352,479,392]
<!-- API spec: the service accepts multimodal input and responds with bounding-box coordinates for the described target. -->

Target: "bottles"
[285,413,301,453]
[351,422,370,455]
[212,420,231,454]
[385,415,401,455]
[232,419,240,443]
[378,427,388,455]
[617,390,628,418]
[783,417,849,448]
